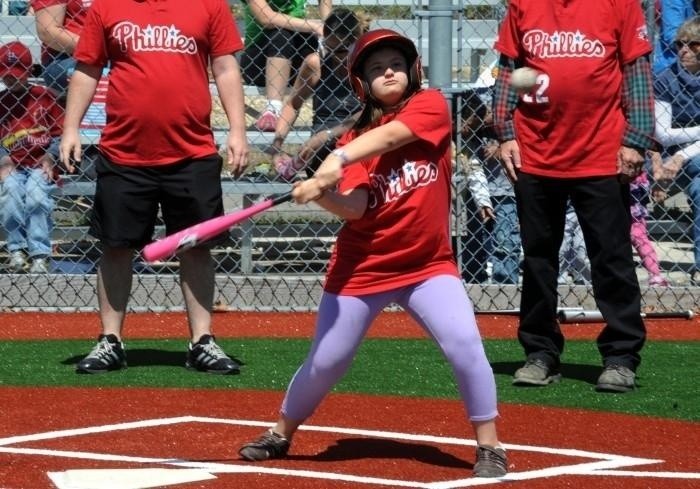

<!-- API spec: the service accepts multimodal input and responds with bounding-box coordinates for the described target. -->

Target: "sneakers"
[596,361,637,393]
[76,332,128,374]
[10,250,27,270]
[690,268,700,287]
[255,105,282,133]
[472,441,508,480]
[648,275,670,287]
[238,426,291,462]
[185,334,241,375]
[511,354,563,387]
[29,258,48,274]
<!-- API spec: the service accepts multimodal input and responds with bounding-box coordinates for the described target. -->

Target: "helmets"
[347,28,422,103]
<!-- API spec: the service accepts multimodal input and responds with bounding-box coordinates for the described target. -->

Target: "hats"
[0,41,32,83]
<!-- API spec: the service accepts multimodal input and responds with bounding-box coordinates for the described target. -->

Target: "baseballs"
[510,68,538,94]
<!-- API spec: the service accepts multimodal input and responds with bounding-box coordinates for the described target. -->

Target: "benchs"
[0,15,700,286]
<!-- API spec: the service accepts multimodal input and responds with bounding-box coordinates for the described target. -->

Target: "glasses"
[322,41,350,54]
[673,39,700,53]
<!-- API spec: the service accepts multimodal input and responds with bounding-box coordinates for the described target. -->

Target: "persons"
[238,28,508,477]
[492,0,654,391]
[239,1,668,287]
[59,0,249,374]
[652,1,700,286]
[1,1,92,270]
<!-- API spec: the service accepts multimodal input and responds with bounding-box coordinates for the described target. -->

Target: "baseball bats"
[142,192,293,262]
